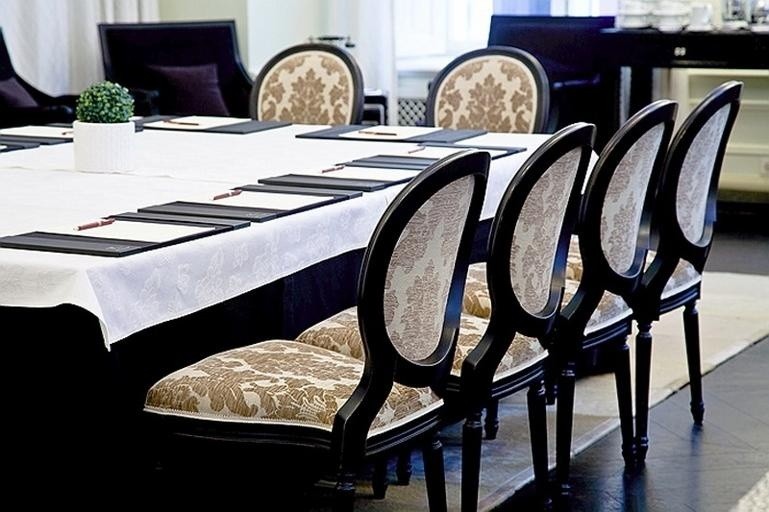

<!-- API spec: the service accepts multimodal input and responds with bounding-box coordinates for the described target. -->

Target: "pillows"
[145,58,231,120]
[1,73,39,112]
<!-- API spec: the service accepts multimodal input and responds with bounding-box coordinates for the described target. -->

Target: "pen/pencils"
[408,148,424,153]
[213,191,241,200]
[166,120,198,125]
[320,165,344,173]
[73,218,115,230]
[358,131,396,135]
[63,130,73,135]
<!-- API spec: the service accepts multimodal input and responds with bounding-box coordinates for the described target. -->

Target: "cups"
[619,3,714,35]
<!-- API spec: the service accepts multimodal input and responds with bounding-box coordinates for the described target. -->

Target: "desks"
[0,111,602,510]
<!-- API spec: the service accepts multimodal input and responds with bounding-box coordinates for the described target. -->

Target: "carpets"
[305,262,767,509]
[726,467,769,509]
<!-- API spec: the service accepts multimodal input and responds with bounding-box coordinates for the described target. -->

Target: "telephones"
[310,35,355,48]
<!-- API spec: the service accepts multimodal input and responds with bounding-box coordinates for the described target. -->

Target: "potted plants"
[70,78,140,173]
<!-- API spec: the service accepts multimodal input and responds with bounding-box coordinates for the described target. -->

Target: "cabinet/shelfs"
[669,70,769,194]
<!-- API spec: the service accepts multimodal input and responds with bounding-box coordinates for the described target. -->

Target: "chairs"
[293,114,607,509]
[97,20,389,129]
[563,78,750,473]
[1,23,86,123]
[422,41,555,138]
[422,12,625,151]
[461,99,680,508]
[137,144,492,512]
[248,41,369,131]
